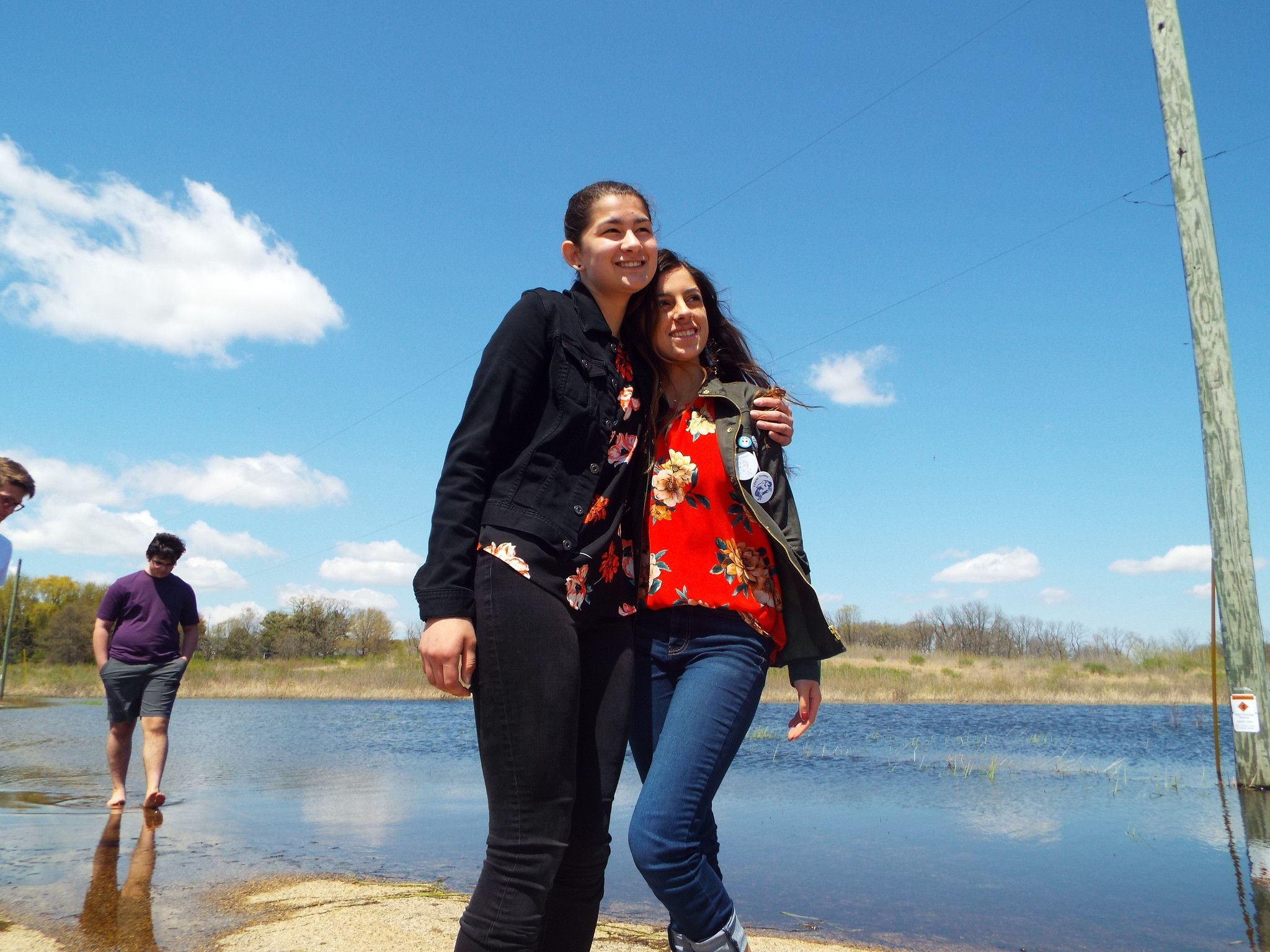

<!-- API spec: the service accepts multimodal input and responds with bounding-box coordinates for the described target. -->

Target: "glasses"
[152,559,177,569]
[3,496,25,512]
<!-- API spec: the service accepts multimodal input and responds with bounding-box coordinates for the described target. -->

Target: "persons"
[78,799,163,952]
[94,533,200,807]
[0,457,35,586]
[413,184,794,952]
[562,248,848,952]
